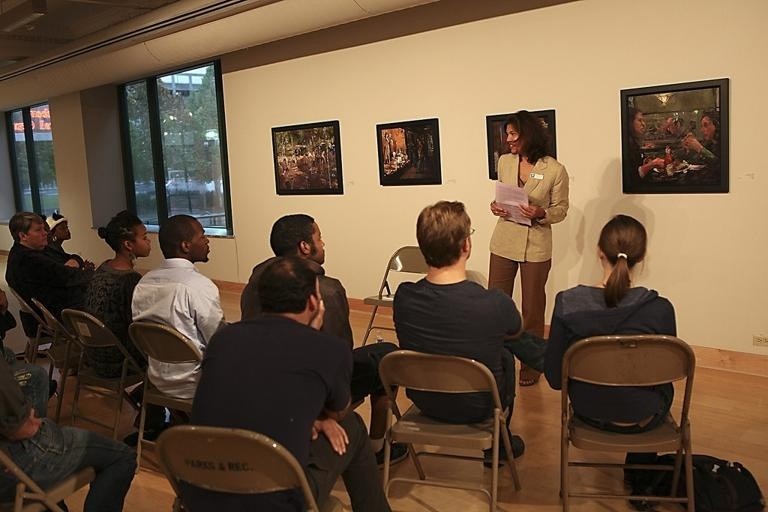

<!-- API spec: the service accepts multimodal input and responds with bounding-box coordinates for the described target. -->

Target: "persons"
[174,255,396,512]
[239,213,409,468]
[2,358,137,510]
[129,215,233,401]
[628,106,721,185]
[5,212,95,377]
[82,211,167,429]
[489,110,569,385]
[393,201,549,469]
[545,214,679,433]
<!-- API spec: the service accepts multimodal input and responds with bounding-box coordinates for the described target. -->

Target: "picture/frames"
[486,109,556,181]
[620,78,732,194]
[271,120,344,196]
[376,118,442,186]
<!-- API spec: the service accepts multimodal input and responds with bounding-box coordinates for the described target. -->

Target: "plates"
[653,165,691,174]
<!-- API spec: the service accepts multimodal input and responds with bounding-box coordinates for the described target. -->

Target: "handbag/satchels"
[651,453,765,512]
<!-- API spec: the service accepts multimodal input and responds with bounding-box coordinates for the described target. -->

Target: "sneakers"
[374,440,410,470]
[484,433,525,468]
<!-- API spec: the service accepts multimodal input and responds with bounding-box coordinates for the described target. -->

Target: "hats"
[43,212,68,231]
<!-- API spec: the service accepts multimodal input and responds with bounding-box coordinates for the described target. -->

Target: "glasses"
[458,228,475,236]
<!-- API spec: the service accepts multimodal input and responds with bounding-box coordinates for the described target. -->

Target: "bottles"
[665,145,673,164]
[375,331,385,344]
